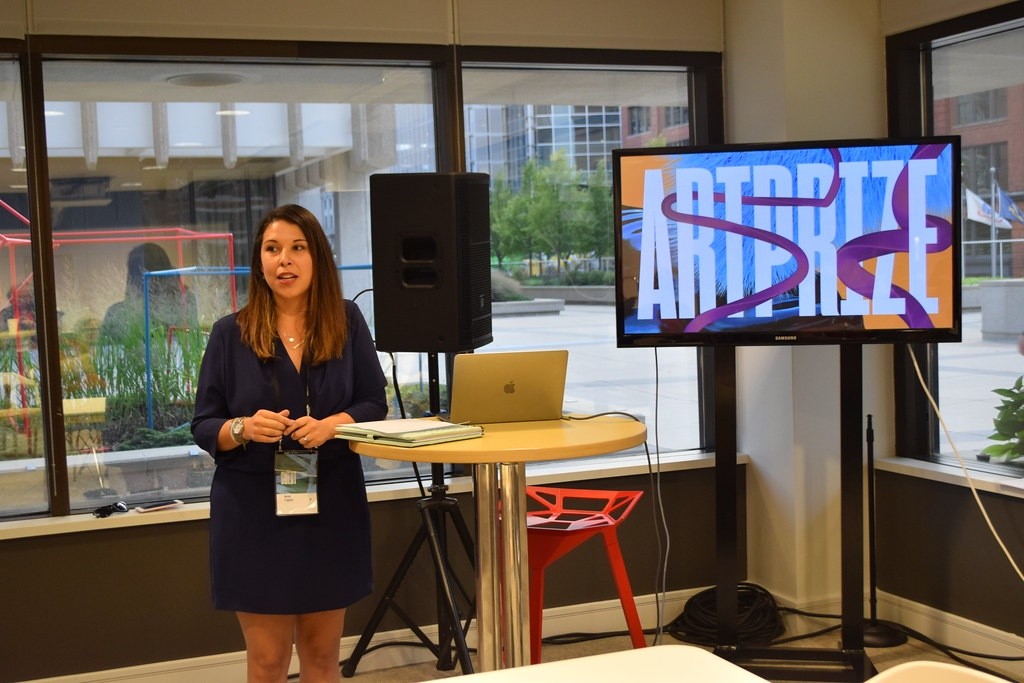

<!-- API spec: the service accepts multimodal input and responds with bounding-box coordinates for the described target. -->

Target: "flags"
[996,182,1024,224]
[966,188,1013,229]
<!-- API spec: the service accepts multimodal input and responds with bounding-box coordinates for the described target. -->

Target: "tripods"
[341,352,479,678]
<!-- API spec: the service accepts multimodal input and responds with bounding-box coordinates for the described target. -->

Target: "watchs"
[232,416,250,445]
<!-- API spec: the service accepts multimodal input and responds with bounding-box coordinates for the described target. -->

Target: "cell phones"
[135,499,184,513]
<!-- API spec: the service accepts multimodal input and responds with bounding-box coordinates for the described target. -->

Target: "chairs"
[500,485,644,665]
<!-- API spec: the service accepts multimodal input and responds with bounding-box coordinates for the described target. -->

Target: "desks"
[349,418,650,671]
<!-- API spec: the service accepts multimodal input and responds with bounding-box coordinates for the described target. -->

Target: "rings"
[302,437,309,443]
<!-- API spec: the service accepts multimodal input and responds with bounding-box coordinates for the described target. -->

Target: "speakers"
[367,172,492,353]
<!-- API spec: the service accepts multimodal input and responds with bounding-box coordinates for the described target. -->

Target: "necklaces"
[276,326,305,349]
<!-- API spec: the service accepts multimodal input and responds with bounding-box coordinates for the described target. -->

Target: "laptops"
[438,350,569,424]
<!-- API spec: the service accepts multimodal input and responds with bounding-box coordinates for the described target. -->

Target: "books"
[334,418,483,447]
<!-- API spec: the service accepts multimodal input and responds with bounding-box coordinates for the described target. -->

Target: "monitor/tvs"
[610,135,961,349]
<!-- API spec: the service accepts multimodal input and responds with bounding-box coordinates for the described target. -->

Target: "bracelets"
[229,417,240,445]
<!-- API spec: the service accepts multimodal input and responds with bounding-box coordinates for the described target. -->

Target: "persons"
[95,242,209,495]
[191,204,389,683]
[0,284,101,432]
